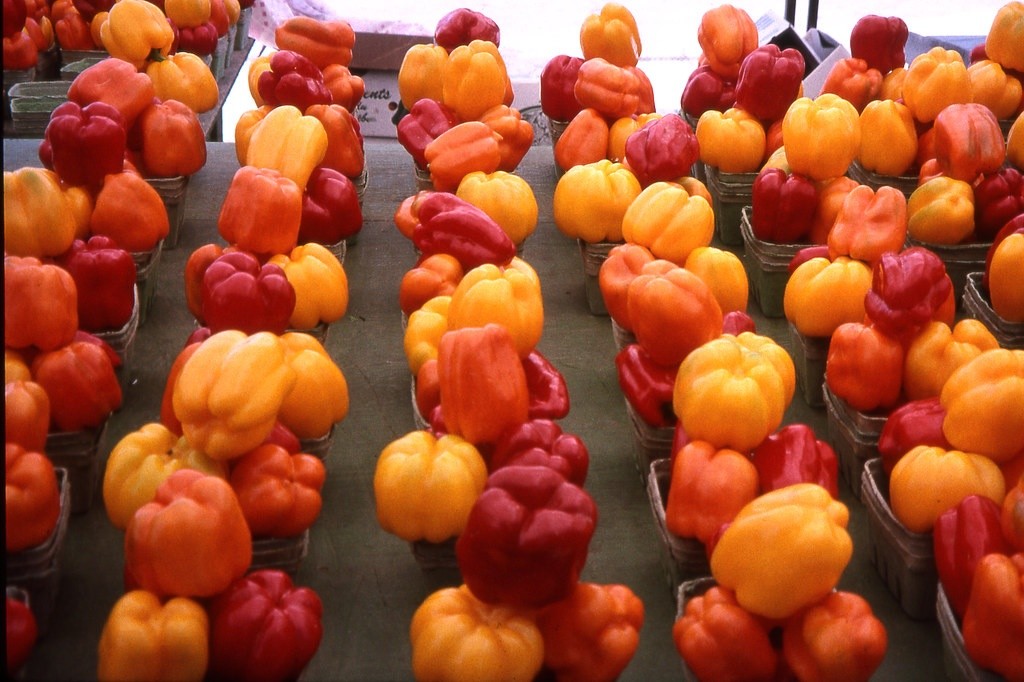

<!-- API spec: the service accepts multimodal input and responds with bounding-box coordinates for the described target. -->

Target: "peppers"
[5,0,1024,682]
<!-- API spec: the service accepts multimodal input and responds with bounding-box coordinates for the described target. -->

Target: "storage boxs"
[0,0,1024,682]
